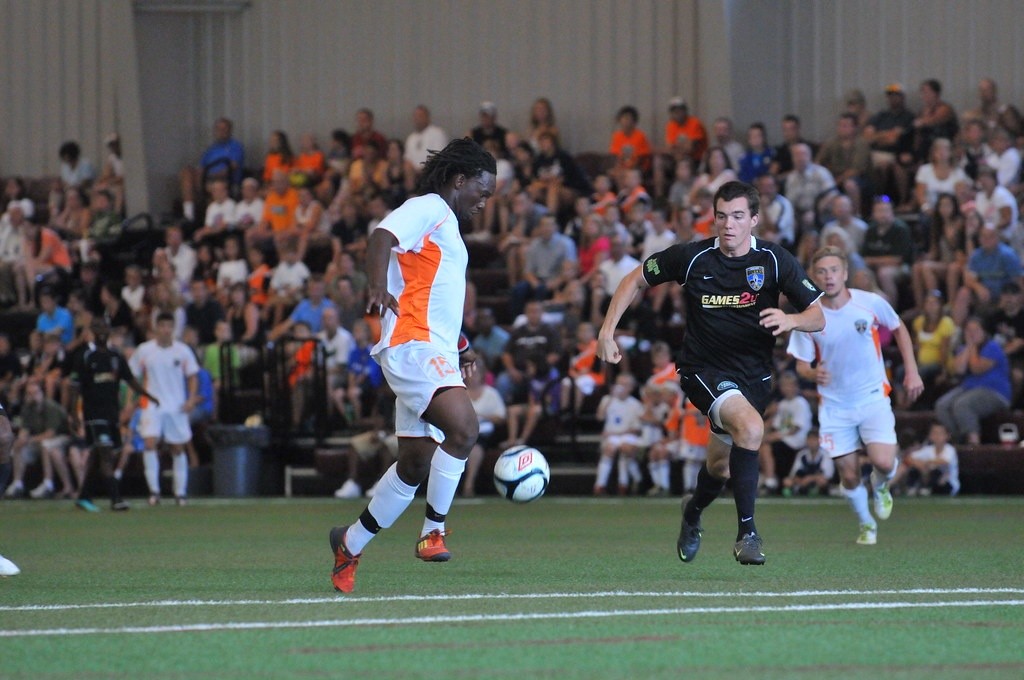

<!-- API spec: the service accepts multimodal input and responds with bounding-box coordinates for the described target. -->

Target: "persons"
[330,137,497,592]
[594,181,825,566]
[0,77,1024,515]
[785,246,924,545]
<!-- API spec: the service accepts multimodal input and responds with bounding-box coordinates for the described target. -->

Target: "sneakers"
[416,528,451,561]
[857,522,877,545]
[30,484,53,499]
[329,525,361,594]
[870,471,893,520]
[0,554,21,578]
[110,501,130,512]
[335,479,361,499]
[75,498,103,513]
[4,486,27,499]
[676,494,704,562]
[733,535,766,566]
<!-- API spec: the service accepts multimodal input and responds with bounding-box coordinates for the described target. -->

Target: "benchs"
[84,214,1024,497]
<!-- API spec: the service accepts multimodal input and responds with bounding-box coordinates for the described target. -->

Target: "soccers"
[493,444,551,505]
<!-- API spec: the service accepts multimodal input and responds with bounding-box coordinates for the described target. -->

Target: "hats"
[667,98,689,111]
[478,101,500,117]
[884,86,905,98]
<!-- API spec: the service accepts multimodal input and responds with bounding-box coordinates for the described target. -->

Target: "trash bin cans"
[203,425,271,497]
